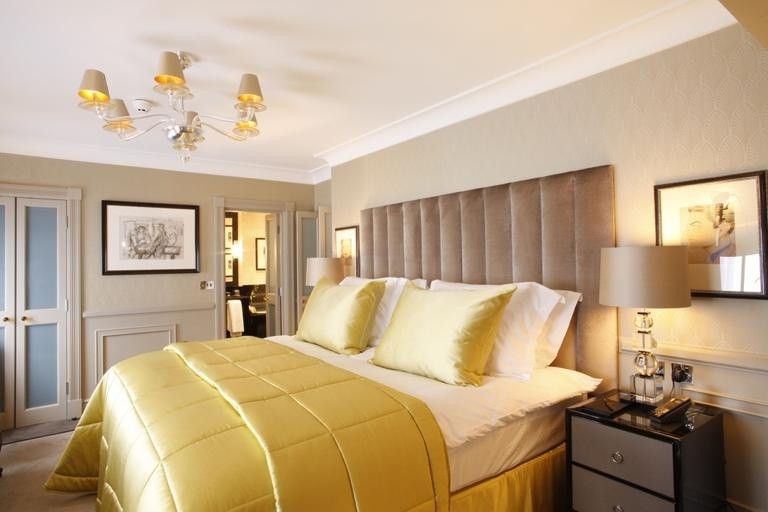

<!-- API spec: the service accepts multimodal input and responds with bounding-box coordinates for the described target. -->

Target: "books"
[579,398,630,417]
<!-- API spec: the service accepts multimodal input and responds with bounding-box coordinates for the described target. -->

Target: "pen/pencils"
[602,398,614,411]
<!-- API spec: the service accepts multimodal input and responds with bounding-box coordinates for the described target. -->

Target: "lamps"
[597,243,693,409]
[75,48,269,164]
[305,256,346,288]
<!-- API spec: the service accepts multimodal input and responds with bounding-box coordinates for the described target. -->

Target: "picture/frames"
[652,169,768,300]
[101,200,202,276]
[255,237,268,271]
[224,253,233,278]
[334,223,360,277]
[224,224,233,249]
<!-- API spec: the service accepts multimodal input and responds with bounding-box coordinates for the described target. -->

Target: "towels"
[226,299,245,337]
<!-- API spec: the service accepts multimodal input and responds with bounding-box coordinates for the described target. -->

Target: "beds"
[47,161,618,511]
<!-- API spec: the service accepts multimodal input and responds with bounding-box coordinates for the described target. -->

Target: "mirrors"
[224,211,240,288]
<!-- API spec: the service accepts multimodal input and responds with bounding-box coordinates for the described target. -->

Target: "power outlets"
[669,361,694,387]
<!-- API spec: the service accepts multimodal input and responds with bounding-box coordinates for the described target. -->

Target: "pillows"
[338,276,410,348]
[533,290,584,371]
[429,279,566,383]
[365,279,519,388]
[295,274,387,356]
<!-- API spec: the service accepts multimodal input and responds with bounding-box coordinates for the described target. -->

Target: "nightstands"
[563,388,729,511]
